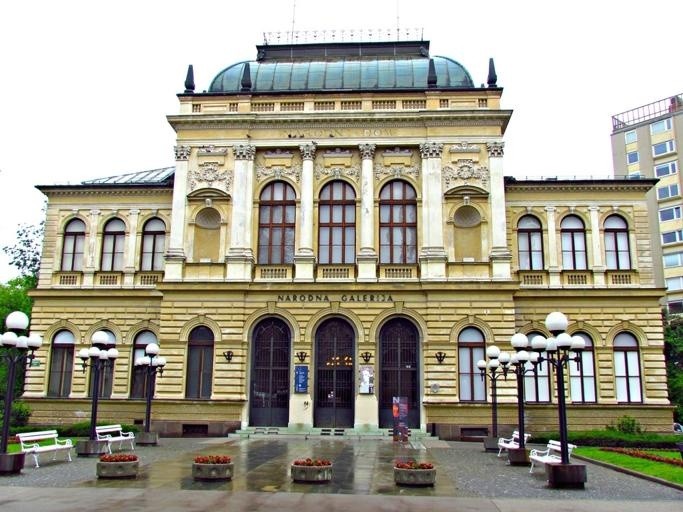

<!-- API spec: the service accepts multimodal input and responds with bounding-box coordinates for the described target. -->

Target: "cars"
[672,421,683,434]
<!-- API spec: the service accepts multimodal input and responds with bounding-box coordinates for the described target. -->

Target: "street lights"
[0,311,42,474]
[75,331,118,456]
[133,343,166,445]
[498,331,538,463]
[476,346,510,452]
[531,311,588,489]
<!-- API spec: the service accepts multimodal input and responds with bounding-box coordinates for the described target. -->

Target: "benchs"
[16,429,74,468]
[529,439,577,482]
[496,430,531,462]
[94,424,136,454]
